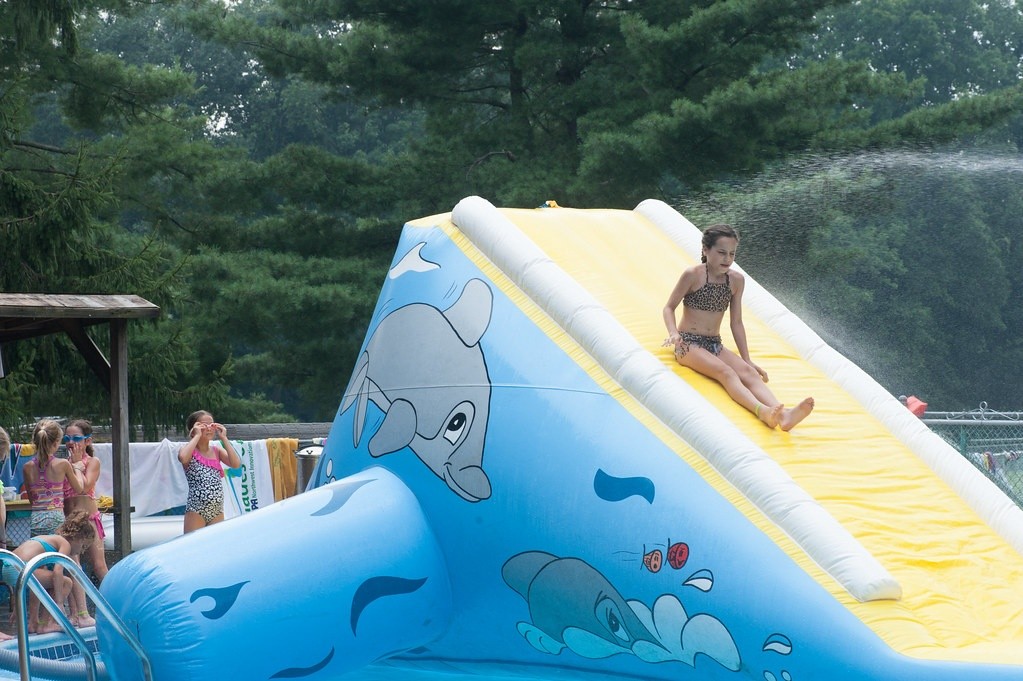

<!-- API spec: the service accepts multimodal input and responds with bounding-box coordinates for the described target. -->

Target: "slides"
[444,196,1022,674]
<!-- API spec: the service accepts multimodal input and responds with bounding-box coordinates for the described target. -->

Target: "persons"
[662,225,814,432]
[0,426,15,642]
[60,419,108,627]
[22,418,96,629]
[178,410,241,533]
[3,509,95,635]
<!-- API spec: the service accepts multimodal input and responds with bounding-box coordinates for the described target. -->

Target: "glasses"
[63,433,91,442]
[189,423,217,439]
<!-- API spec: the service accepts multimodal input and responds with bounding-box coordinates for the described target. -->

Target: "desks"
[5,498,31,511]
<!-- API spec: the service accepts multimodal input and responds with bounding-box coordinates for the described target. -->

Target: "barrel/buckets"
[292,446,322,495]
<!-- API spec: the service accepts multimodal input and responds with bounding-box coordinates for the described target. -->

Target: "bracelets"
[75,467,82,472]
